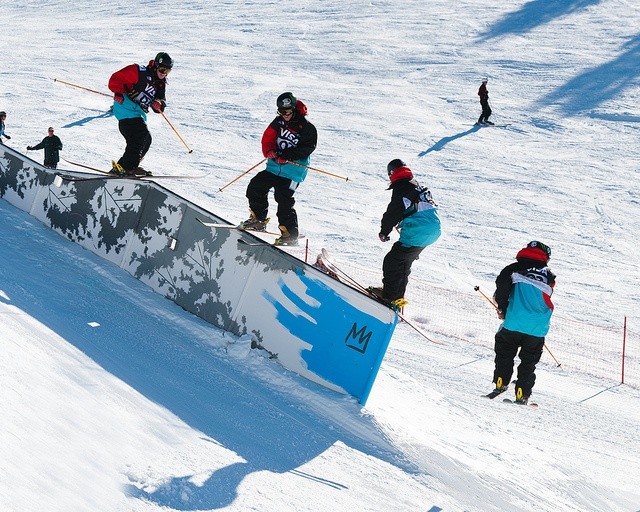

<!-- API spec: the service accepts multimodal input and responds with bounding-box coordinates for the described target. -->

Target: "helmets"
[154,52,173,70]
[0,111,6,116]
[387,159,406,174]
[482,77,488,82]
[527,241,551,260]
[277,92,297,109]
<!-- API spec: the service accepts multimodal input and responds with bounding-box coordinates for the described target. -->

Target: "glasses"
[157,67,171,74]
[277,110,295,116]
[2,115,6,118]
[48,129,54,132]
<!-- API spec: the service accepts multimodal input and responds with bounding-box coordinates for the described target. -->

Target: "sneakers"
[372,287,403,305]
[485,120,492,125]
[133,167,152,176]
[478,120,485,124]
[496,376,508,391]
[275,226,298,245]
[114,164,138,176]
[242,209,270,230]
[516,388,528,403]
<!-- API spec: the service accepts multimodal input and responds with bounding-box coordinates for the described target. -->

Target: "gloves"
[0,138,3,143]
[114,93,125,104]
[152,99,161,111]
[27,146,32,150]
[267,150,278,160]
[277,149,291,165]
[379,232,390,242]
[5,135,11,139]
[497,307,505,319]
[57,145,62,150]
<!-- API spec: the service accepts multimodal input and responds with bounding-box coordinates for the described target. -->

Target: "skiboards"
[195,217,305,246]
[63,159,202,180]
[482,380,538,406]
[463,124,511,127]
[321,248,444,345]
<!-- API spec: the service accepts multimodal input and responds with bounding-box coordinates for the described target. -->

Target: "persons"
[477,77,496,125]
[0,112,11,146]
[27,127,64,170]
[488,241,556,407]
[367,158,442,312]
[240,92,317,245]
[107,53,174,178]
